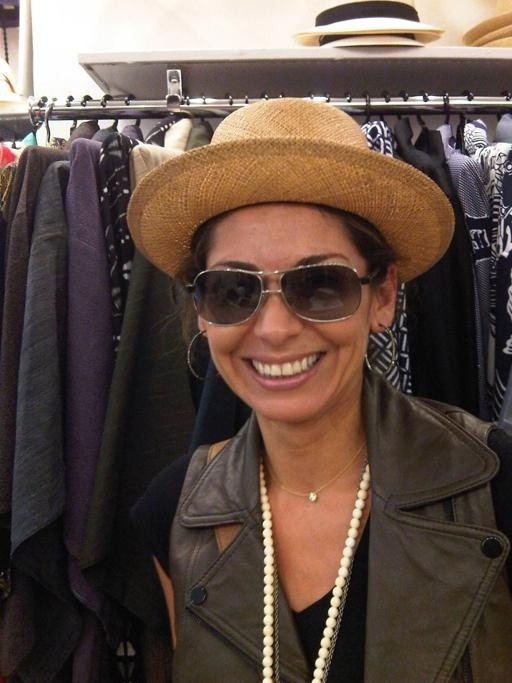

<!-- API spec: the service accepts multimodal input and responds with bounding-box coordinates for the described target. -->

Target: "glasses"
[185,263,384,327]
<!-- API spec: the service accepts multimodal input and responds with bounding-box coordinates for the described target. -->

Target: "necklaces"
[263,438,368,502]
[260,463,371,683]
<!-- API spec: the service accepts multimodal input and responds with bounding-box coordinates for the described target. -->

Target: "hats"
[294,1,512,49]
[124,97,456,279]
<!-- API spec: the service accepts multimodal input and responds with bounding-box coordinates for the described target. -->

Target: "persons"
[126,96,512,683]
